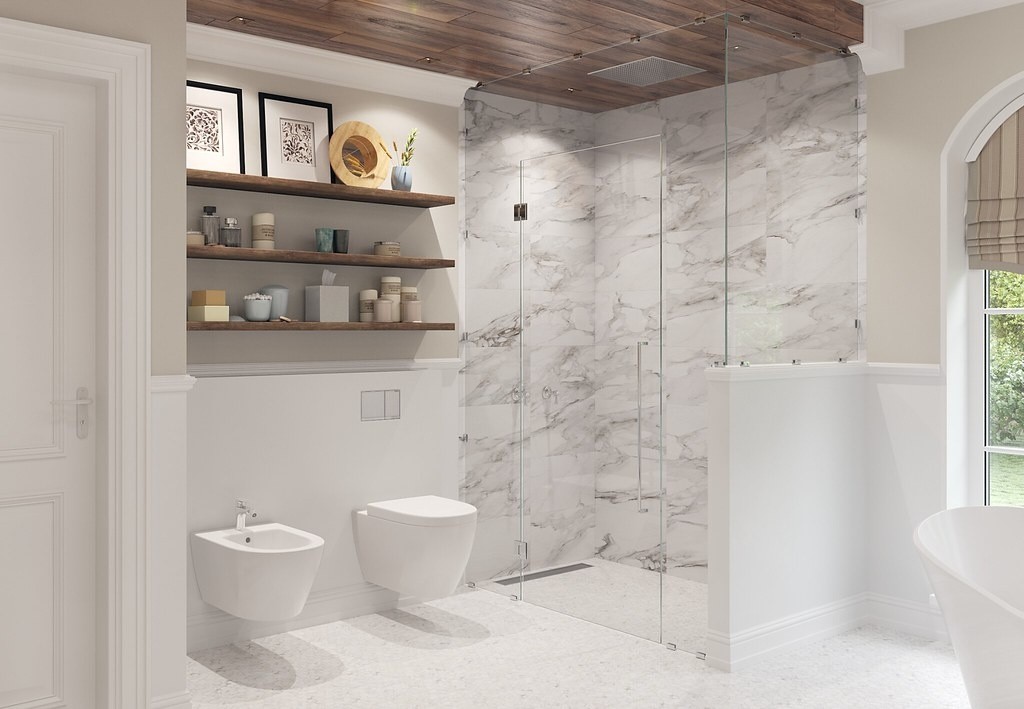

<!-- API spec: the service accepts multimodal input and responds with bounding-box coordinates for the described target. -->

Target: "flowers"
[379,128,418,166]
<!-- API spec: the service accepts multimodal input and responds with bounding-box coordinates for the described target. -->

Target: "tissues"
[304,269,350,322]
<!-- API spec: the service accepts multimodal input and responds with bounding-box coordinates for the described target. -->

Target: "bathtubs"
[910,504,1024,709]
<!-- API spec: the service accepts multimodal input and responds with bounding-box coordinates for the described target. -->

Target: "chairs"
[912,502,1022,707]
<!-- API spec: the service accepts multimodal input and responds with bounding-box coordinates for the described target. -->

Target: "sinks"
[190,522,325,624]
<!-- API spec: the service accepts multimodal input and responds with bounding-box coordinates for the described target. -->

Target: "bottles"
[374,241,400,256]
[243,299,271,321]
[359,289,378,321]
[260,284,290,322]
[372,298,393,323]
[401,300,422,322]
[400,286,417,323]
[220,217,241,247]
[391,165,412,192]
[379,276,401,323]
[200,205,221,246]
[251,212,274,249]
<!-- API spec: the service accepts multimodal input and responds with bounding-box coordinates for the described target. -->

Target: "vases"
[391,166,412,192]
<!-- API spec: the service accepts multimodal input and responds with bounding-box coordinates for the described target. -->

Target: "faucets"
[233,499,258,533]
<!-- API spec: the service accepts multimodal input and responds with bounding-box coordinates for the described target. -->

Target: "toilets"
[350,494,477,601]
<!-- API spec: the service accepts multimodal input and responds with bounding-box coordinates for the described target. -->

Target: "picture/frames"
[185,79,244,175]
[257,91,336,183]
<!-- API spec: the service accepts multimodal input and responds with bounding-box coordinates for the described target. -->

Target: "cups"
[333,229,350,253]
[315,228,333,253]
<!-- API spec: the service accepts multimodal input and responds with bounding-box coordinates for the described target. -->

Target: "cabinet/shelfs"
[186,167,458,330]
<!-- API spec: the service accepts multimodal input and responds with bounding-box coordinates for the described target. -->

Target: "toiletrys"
[184,205,422,323]
